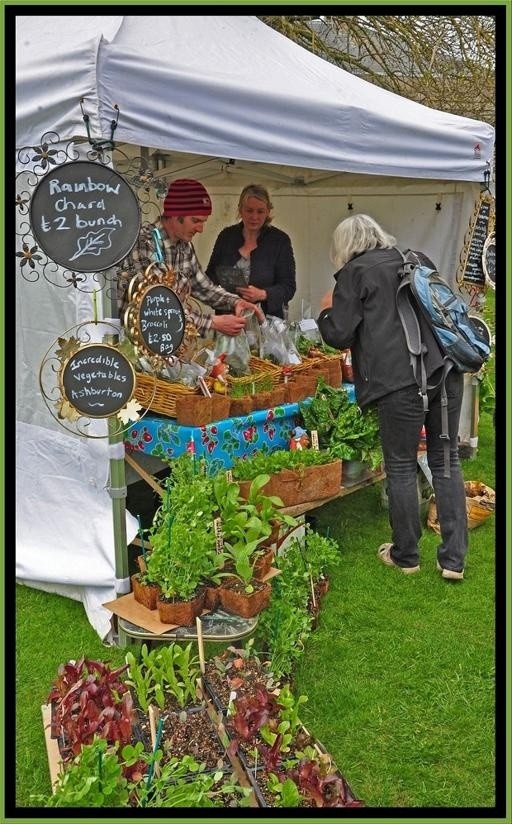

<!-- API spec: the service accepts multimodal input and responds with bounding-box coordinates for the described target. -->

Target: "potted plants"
[227,444,344,508]
[131,450,300,628]
[174,370,320,428]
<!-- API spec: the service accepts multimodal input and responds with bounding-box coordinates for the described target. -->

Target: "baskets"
[132,344,344,426]
[238,457,343,512]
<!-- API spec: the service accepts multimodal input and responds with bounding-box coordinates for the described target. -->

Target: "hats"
[163,178,212,217]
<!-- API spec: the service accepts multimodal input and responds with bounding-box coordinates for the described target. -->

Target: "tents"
[15,14,495,635]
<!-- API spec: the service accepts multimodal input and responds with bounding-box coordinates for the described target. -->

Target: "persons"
[115,177,266,340]
[314,209,473,587]
[206,182,298,323]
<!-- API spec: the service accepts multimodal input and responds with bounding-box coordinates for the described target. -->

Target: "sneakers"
[436,559,465,580]
[377,542,420,575]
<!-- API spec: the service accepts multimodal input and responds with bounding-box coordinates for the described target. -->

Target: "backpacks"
[393,245,491,374]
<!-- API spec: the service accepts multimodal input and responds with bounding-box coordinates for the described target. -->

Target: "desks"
[117,592,260,665]
[122,383,427,552]
